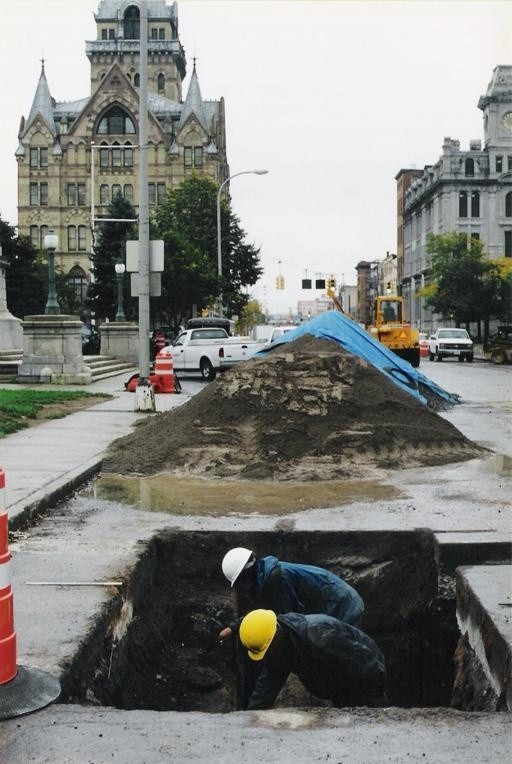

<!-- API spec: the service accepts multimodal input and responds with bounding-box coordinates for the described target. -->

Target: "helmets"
[221,548,254,588]
[240,608,277,661]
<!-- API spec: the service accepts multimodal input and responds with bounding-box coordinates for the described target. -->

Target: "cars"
[266,326,296,345]
[484,331,511,364]
[428,327,474,363]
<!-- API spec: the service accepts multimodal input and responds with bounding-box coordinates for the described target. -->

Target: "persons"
[152,328,165,355]
[217,546,367,694]
[239,610,396,711]
[383,302,395,321]
[90,326,98,354]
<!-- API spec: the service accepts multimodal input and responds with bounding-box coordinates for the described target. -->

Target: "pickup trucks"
[159,327,267,381]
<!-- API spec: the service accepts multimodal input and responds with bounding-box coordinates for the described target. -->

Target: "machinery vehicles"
[368,295,420,368]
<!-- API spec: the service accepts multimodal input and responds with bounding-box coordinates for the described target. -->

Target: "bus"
[187,317,235,336]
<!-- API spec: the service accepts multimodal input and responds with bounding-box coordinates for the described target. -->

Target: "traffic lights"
[276,277,284,290]
[329,277,336,287]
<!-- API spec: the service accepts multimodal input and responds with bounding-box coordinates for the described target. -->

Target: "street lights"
[45,235,60,315]
[216,170,268,316]
[115,264,125,321]
